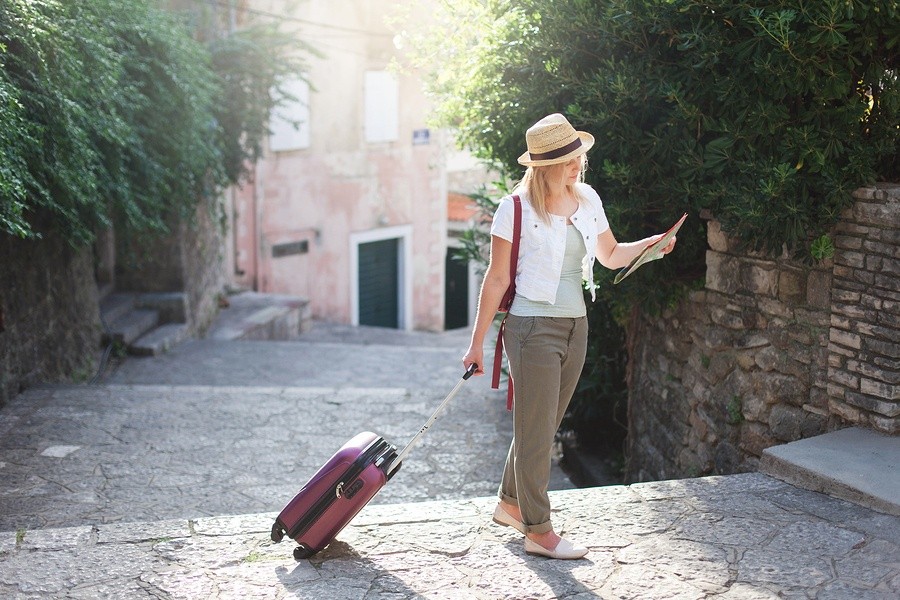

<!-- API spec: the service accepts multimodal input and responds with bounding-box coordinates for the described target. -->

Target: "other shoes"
[492,501,528,535]
[524,531,592,561]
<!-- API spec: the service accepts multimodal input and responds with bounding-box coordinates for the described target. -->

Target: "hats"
[517,112,597,166]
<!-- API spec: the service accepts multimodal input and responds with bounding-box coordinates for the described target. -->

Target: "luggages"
[270,360,479,561]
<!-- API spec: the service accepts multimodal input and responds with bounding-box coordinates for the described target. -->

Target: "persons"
[463,114,676,559]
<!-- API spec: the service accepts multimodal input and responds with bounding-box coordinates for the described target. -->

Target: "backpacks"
[489,194,523,412]
